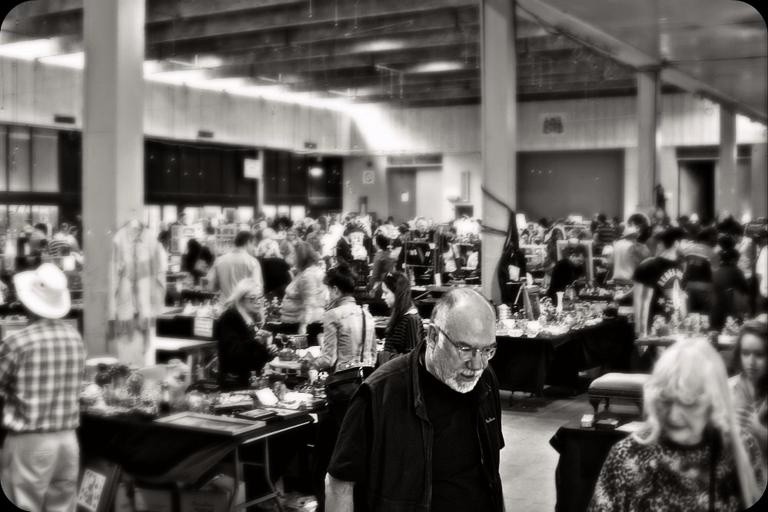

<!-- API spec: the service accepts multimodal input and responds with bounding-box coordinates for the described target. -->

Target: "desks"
[372,300,639,410]
[64,351,366,511]
[550,410,671,512]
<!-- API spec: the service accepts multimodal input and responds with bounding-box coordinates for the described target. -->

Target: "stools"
[587,372,648,416]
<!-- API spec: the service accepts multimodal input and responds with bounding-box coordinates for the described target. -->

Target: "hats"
[12,262,72,320]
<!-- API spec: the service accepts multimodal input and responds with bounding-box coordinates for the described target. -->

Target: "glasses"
[437,327,496,362]
[245,293,265,303]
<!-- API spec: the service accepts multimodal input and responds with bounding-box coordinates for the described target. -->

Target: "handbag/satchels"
[325,371,362,402]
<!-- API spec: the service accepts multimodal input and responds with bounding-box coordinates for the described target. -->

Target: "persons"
[321,278,508,510]
[519,209,767,492]
[310,264,379,512]
[587,333,764,511]
[164,208,478,394]
[0,264,88,510]
[1,218,84,299]
